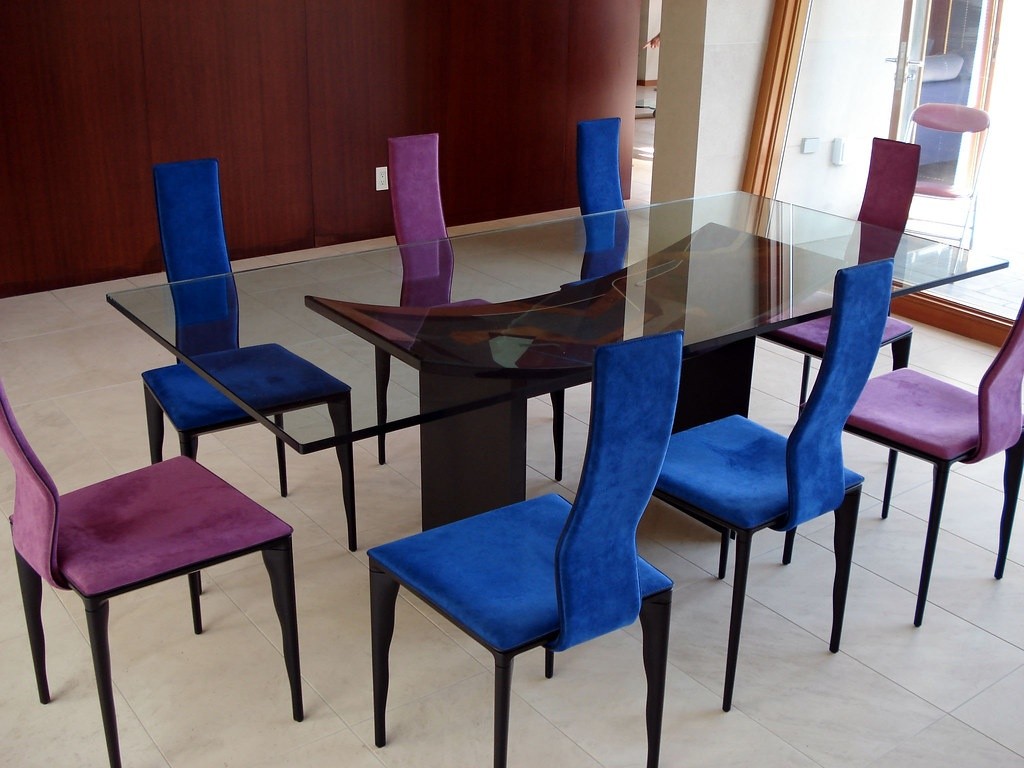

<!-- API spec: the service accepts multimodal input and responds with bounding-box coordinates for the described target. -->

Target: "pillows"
[922,53,964,82]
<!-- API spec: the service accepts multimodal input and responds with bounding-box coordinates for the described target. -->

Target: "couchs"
[914,48,974,166]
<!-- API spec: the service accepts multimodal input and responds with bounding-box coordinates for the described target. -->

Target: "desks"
[100,191,1013,531]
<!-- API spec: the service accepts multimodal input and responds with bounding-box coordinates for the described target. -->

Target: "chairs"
[366,328,686,768]
[561,118,630,290]
[140,157,357,597]
[374,133,567,485]
[651,256,896,714]
[844,101,989,286]
[800,292,1024,630]
[754,136,922,412]
[0,379,306,768]
